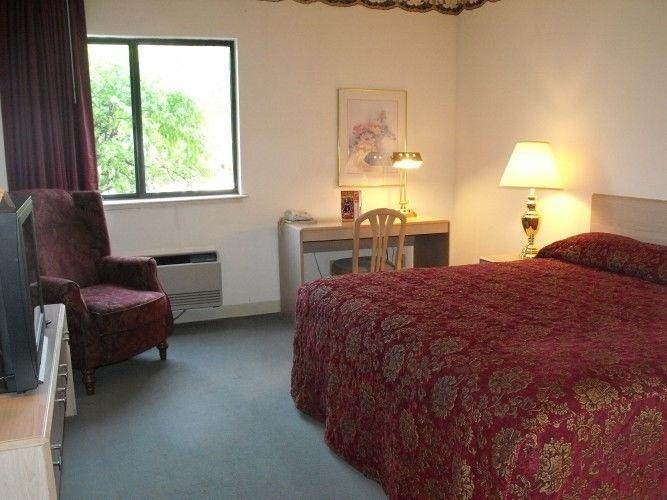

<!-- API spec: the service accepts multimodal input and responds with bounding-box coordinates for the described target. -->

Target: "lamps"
[495,141,566,261]
[388,150,423,217]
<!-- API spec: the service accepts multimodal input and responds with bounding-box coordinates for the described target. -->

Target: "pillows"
[533,229,667,286]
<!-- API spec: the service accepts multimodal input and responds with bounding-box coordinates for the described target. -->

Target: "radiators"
[146,250,224,314]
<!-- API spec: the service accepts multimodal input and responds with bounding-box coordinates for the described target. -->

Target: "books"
[340,189,361,222]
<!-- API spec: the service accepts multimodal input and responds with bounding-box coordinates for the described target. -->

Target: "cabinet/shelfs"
[0,302,80,499]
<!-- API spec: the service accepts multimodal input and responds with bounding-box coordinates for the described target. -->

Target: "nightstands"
[475,253,519,267]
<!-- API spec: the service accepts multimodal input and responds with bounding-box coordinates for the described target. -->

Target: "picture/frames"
[333,83,408,190]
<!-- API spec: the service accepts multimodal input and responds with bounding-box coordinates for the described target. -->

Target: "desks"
[277,210,451,331]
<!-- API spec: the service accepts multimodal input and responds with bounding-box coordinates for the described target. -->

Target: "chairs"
[328,207,407,278]
[4,187,175,397]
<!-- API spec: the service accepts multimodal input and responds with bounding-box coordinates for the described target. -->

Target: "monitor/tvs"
[0,191,48,394]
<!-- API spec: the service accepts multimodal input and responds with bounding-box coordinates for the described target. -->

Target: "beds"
[288,189,667,499]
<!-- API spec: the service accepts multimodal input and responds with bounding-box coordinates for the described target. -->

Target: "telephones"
[284,208,313,222]
